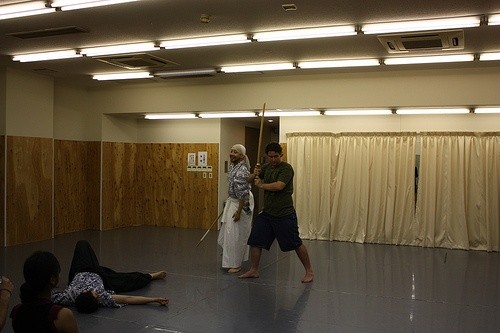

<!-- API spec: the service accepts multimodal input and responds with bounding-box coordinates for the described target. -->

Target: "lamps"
[0,0,500,120]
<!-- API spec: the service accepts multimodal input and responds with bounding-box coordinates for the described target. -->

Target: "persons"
[238,143,313,283]
[0,276,14,329]
[217,144,254,273]
[9,250,79,333]
[51,240,168,314]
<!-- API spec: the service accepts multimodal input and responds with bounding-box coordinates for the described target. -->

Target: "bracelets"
[0,289,12,296]
